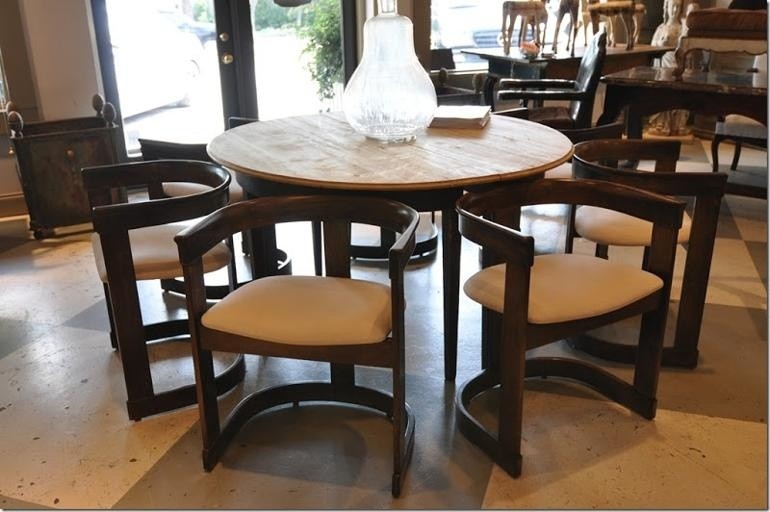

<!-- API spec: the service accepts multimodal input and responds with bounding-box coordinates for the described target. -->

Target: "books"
[430,105,493,128]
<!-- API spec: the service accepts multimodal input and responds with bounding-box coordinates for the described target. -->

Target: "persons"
[651,1,682,131]
